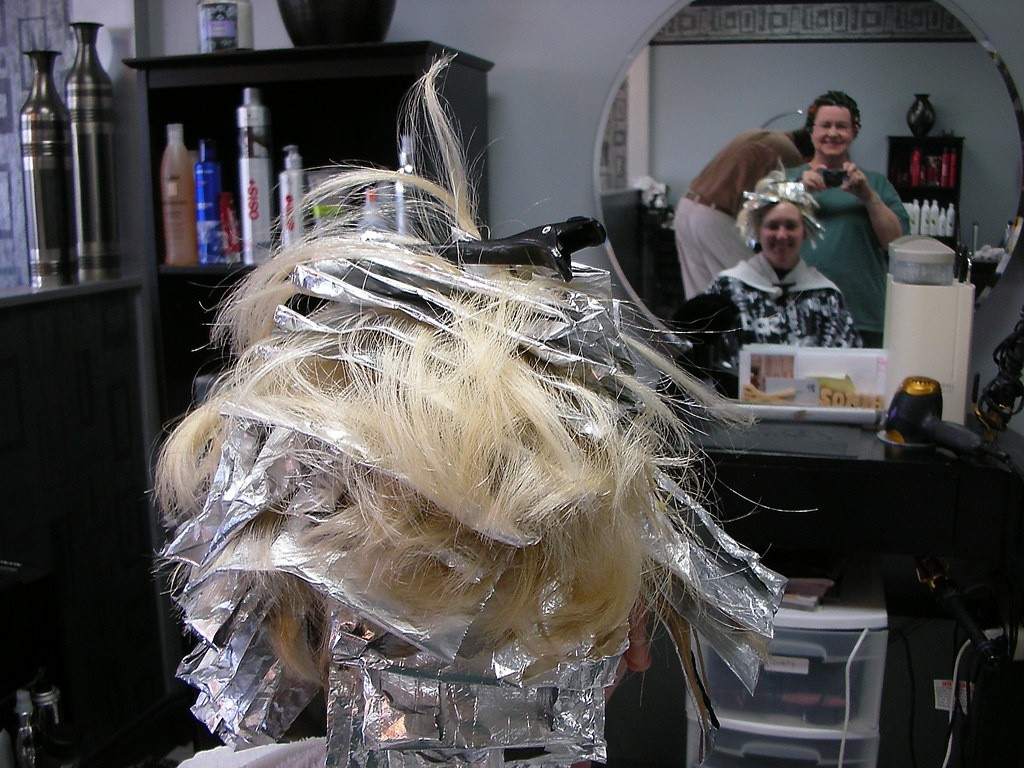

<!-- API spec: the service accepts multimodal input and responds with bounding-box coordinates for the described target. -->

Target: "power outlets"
[998,601,1024,663]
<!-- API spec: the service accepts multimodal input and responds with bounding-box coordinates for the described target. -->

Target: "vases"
[906,94,935,136]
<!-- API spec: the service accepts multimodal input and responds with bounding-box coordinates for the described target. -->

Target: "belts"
[685,193,735,217]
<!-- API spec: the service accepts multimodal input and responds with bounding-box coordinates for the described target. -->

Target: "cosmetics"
[161,87,416,266]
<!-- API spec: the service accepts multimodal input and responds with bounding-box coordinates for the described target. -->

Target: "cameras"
[822,169,849,188]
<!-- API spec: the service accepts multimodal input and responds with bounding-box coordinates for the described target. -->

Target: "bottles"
[161,123,196,265]
[911,199,955,236]
[394,135,419,237]
[237,87,275,264]
[942,147,956,186]
[196,138,225,263]
[358,189,387,234]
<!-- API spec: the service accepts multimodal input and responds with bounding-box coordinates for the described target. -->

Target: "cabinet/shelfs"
[1,276,143,768]
[123,41,496,449]
[886,134,965,250]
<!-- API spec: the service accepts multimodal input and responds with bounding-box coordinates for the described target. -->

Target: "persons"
[675,128,816,298]
[153,52,755,768]
[705,171,863,348]
[766,92,911,333]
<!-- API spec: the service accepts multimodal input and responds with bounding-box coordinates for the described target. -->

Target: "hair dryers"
[885,378,989,462]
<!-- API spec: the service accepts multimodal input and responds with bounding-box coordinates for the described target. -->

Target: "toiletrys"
[895,145,957,188]
[901,199,955,237]
[195,0,255,54]
[23,23,420,292]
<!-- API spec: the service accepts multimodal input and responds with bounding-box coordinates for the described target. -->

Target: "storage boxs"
[681,568,888,768]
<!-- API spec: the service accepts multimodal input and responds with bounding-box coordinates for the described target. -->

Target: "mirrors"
[594,1,1023,395]
